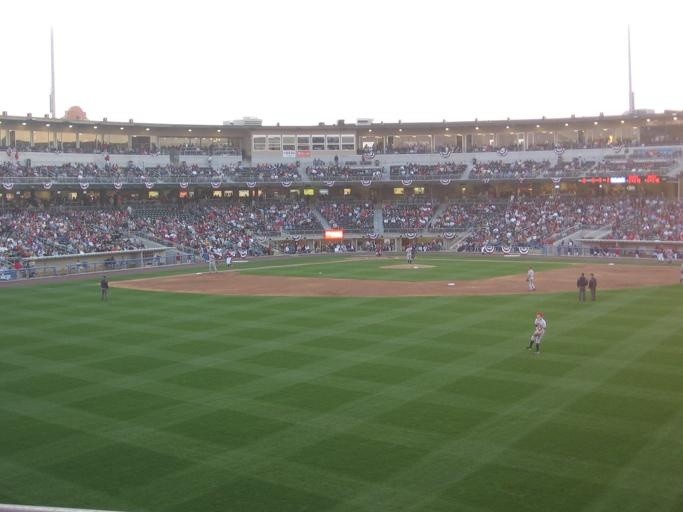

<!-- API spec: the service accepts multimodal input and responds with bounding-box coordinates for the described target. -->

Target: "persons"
[526,311,547,354]
[526,267,536,292]
[588,272,598,301]
[100,275,109,301]
[576,272,588,303]
